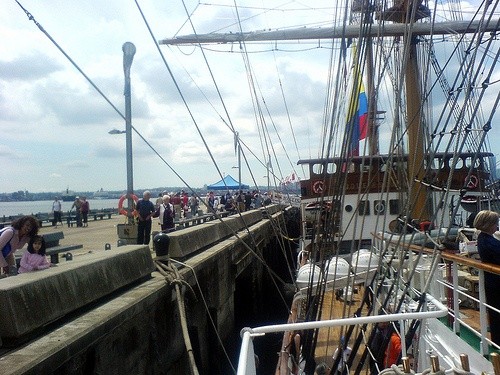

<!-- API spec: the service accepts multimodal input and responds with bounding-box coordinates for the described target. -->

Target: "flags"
[341,42,369,173]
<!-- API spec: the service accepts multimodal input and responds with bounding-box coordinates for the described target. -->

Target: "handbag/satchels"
[0,228,15,258]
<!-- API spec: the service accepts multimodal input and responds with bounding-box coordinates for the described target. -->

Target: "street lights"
[107,129,134,224]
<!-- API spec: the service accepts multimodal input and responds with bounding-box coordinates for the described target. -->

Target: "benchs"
[15,231,80,270]
[0,209,113,229]
[182,210,231,230]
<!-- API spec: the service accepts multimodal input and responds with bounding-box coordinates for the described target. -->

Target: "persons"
[220,190,272,214]
[152,191,199,233]
[70,195,91,228]
[135,191,156,245]
[52,196,63,229]
[0,216,38,278]
[17,235,50,274]
[473,210,500,351]
[208,191,214,209]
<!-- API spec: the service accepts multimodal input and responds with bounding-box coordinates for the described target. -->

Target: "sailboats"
[17,0,500,375]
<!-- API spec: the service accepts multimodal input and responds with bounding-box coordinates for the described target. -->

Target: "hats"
[264,192,267,194]
[75,196,79,199]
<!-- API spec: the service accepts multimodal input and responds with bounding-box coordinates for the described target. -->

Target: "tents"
[207,175,250,196]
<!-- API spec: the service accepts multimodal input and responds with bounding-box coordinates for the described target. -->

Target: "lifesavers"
[375,204,384,212]
[464,175,478,189]
[313,181,326,194]
[119,195,139,216]
